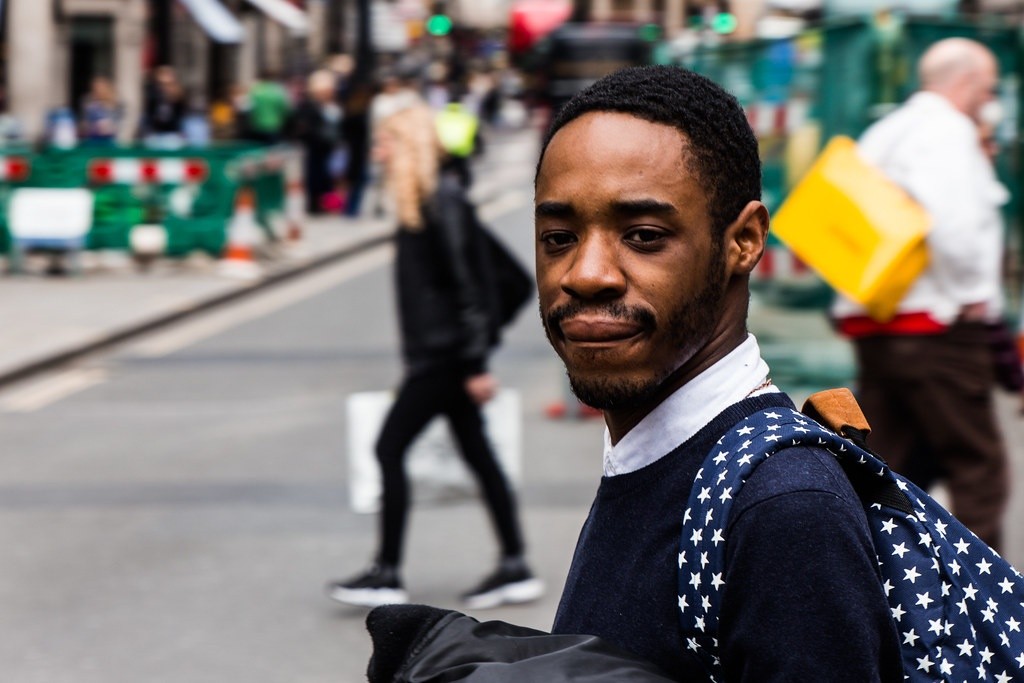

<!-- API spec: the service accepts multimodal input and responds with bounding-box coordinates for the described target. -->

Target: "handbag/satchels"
[347,382,529,515]
[476,218,538,329]
[770,134,933,322]
[676,388,1023,683]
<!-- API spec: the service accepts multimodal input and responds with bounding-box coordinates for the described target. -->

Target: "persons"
[828,38,1011,557]
[371,64,441,225]
[82,55,382,219]
[534,66,904,683]
[327,89,548,609]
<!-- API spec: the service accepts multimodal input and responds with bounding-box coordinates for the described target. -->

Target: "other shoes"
[328,566,407,611]
[458,559,547,609]
